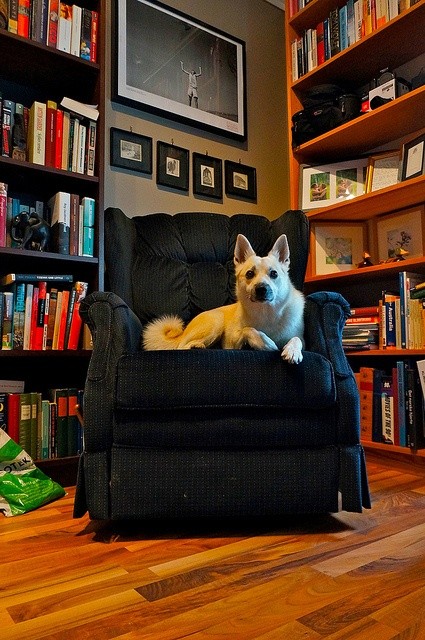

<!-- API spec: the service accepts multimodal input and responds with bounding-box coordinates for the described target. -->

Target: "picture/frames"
[225,160,258,204]
[363,150,399,193]
[193,151,224,200]
[310,220,367,277]
[374,205,425,264]
[110,126,152,177]
[111,0,248,143]
[155,139,190,192]
[401,131,425,178]
[298,151,401,214]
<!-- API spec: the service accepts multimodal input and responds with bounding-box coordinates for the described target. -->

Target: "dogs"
[141,234,307,365]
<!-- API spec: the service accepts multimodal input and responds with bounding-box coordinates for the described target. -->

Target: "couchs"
[75,205,370,527]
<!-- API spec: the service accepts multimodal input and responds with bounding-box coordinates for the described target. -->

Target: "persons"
[179,59,202,109]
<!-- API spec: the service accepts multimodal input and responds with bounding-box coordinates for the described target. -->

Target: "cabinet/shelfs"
[284,0,424,457]
[0,0,106,486]
